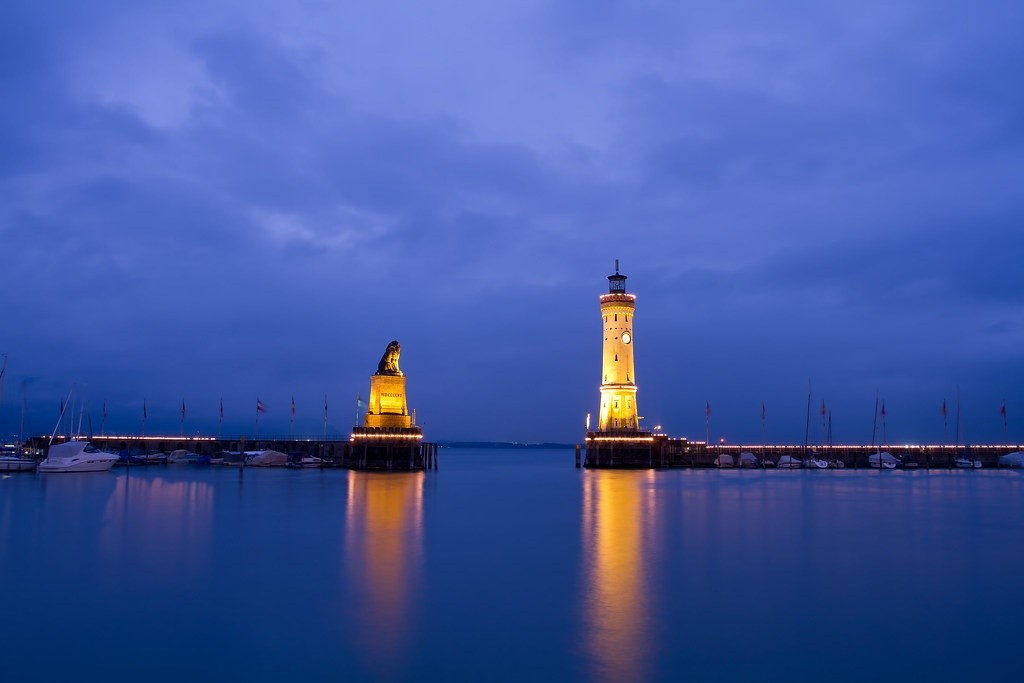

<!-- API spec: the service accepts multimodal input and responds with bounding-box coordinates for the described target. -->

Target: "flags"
[104,399,106,417]
[220,398,224,417]
[324,401,327,420]
[356,397,367,410]
[291,399,295,416]
[257,400,266,412]
[144,398,146,417]
[182,398,185,417]
[60,400,65,417]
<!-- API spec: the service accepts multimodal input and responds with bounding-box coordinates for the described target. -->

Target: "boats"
[131,446,334,468]
[0,455,37,472]
[38,390,120,473]
